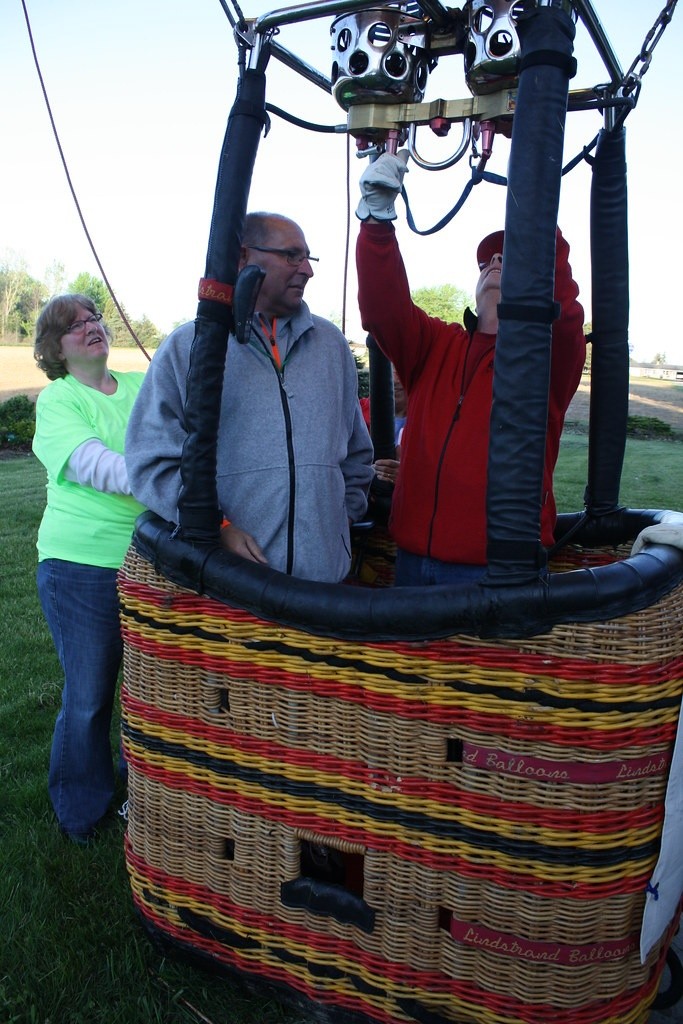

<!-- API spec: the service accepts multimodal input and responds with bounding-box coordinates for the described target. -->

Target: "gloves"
[355,150,413,220]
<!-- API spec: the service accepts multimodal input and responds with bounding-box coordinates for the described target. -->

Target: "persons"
[32,294,146,837]
[372,360,409,491]
[126,213,378,586]
[358,150,586,592]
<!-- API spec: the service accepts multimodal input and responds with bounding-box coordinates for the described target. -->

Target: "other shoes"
[68,831,95,848]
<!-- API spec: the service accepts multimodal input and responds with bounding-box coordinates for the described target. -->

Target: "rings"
[388,474,391,477]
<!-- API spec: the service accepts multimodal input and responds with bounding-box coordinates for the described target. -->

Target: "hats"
[476,229,506,272]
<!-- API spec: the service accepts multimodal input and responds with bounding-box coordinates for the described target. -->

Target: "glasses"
[244,245,320,271]
[62,313,103,334]
